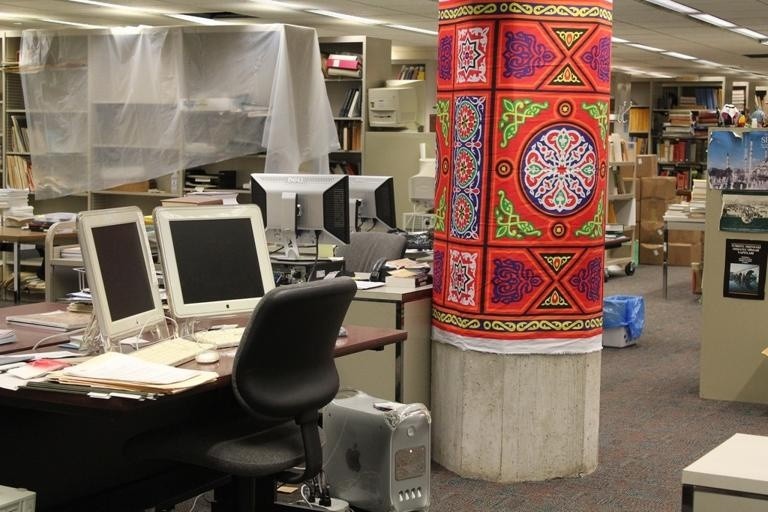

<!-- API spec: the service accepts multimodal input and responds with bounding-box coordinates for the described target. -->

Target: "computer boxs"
[324,386,434,512]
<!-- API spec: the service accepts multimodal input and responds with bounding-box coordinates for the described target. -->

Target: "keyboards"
[192,325,247,349]
[127,336,202,367]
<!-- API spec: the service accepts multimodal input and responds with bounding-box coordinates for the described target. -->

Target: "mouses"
[194,347,220,366]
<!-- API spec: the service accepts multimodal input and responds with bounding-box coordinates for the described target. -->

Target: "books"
[335,87,362,151]
[605,86,767,240]
[378,257,433,292]
[395,64,425,81]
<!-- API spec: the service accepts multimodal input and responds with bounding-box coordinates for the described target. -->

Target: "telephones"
[370,257,398,282]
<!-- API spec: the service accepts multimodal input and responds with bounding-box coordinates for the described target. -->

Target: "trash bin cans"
[603,295,643,348]
[691,262,703,294]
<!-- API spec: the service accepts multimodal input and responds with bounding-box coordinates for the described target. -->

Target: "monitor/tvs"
[151,203,278,332]
[368,78,428,134]
[77,203,164,353]
[249,171,349,266]
[350,174,398,233]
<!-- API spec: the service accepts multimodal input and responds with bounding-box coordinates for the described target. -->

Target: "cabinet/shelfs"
[333,272,431,406]
[0,26,391,306]
[606,75,768,270]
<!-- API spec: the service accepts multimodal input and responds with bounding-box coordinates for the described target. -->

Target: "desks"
[1,302,407,512]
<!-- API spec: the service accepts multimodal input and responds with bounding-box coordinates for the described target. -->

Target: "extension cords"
[274,493,351,512]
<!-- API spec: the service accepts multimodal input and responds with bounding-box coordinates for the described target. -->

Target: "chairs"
[120,281,356,511]
